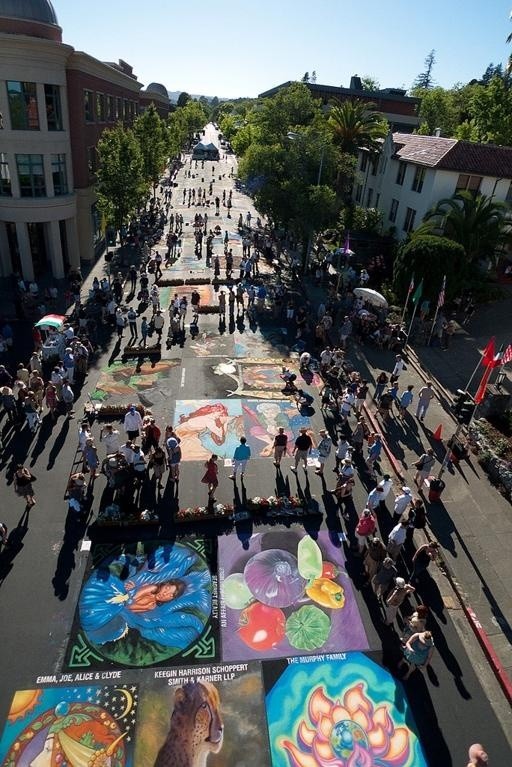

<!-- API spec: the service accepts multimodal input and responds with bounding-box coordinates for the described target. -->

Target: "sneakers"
[228,473,244,479]
[273,461,323,474]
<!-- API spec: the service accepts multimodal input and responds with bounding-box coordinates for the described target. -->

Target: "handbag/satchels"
[30,473,36,482]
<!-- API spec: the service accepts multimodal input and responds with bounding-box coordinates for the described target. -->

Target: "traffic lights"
[457,398,476,425]
[450,388,466,415]
[105,254,111,262]
[109,238,116,244]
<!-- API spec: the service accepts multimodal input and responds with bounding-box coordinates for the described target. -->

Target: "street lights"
[287,131,326,275]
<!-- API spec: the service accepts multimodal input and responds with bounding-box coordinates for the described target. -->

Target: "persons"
[13,463,36,506]
[466,743,488,767]
[111,152,304,280]
[66,404,385,541]
[1,523,8,545]
[353,431,468,668]
[1,263,450,434]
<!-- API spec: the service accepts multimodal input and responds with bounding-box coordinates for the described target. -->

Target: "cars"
[212,121,225,145]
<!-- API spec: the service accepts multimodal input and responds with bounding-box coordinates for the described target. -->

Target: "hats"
[340,446,440,647]
[468,743,488,767]
[318,429,328,435]
[0,352,41,395]
[103,424,113,430]
[63,323,87,352]
[166,438,178,448]
[299,427,307,433]
[109,458,118,467]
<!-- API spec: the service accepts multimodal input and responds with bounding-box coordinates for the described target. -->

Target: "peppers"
[306,577,346,608]
[297,534,324,578]
[322,561,350,580]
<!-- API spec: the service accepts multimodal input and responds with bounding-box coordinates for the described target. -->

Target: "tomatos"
[235,603,287,652]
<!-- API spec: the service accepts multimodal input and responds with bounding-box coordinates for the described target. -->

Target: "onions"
[244,549,309,610]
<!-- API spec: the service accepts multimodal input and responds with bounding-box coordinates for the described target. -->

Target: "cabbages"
[286,605,331,651]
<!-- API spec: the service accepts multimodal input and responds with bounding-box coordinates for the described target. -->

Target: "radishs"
[223,573,254,610]
